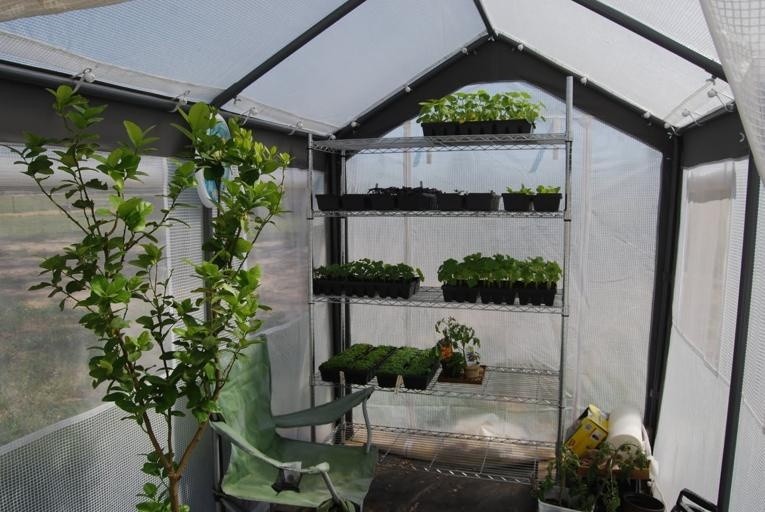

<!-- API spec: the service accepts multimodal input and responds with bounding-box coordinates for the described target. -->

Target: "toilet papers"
[606,404,644,458]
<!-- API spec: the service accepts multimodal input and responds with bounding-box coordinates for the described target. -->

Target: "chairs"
[199,334,380,512]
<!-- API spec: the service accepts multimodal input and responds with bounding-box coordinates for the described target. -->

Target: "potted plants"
[313,190,500,211]
[437,250,561,305]
[500,183,562,212]
[317,344,441,390]
[312,258,425,299]
[534,440,650,512]
[436,316,482,378]
[417,89,546,135]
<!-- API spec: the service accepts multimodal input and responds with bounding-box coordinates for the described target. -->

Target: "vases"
[622,493,665,512]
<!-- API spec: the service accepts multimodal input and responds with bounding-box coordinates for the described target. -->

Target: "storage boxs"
[564,403,609,457]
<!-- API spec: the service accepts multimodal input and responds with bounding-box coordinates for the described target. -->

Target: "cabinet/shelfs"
[307,73,573,485]
